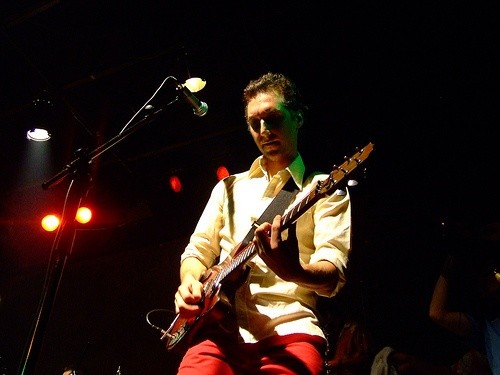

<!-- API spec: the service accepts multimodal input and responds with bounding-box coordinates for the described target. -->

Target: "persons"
[174,73,351,375]
[332,266,500,375]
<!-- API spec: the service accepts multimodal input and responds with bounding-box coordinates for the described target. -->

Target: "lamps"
[174,53,206,93]
[26,107,52,142]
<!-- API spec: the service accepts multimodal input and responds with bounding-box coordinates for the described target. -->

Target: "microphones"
[174,80,208,117]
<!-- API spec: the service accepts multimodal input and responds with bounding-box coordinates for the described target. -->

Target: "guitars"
[161,138,376,354]
